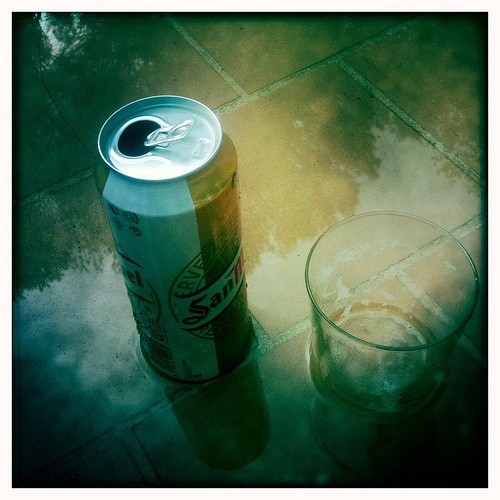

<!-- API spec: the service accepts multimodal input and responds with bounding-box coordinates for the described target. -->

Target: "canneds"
[93,93,253,383]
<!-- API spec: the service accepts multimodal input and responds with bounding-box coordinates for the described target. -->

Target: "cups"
[303,214,480,423]
